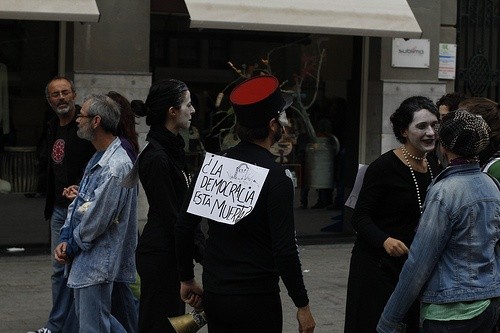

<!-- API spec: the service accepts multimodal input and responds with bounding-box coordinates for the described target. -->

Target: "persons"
[435,92,456,120]
[457,96,500,184]
[53,92,131,332]
[174,71,315,332]
[35,75,97,332]
[54,89,142,332]
[374,107,500,332]
[342,95,445,332]
[129,75,208,332]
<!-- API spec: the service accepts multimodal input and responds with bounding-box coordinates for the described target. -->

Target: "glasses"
[77,114,96,119]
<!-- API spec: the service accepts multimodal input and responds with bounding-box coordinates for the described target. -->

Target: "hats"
[229,74,292,129]
[432,110,491,155]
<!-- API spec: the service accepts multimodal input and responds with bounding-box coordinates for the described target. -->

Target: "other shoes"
[28,327,52,333]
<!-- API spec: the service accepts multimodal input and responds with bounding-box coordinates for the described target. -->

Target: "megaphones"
[168,292,208,333]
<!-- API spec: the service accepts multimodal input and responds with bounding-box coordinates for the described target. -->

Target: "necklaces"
[399,142,435,215]
[166,150,193,189]
